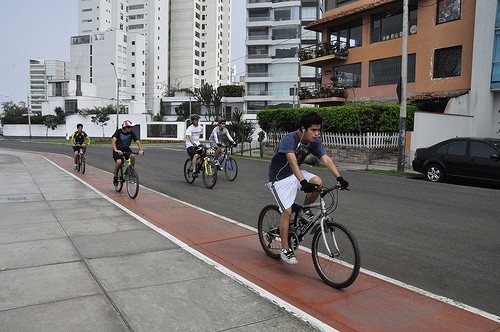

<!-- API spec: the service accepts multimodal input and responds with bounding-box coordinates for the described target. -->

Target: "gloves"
[337,176,348,190]
[300,179,318,193]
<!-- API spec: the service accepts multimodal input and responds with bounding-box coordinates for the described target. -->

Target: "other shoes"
[74,163,76,167]
[214,159,219,165]
[218,166,223,170]
[192,172,197,177]
[113,177,118,186]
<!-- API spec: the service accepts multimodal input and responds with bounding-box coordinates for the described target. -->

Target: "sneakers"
[302,210,318,223]
[281,248,297,264]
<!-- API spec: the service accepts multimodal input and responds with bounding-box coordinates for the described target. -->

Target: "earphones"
[303,129,306,132]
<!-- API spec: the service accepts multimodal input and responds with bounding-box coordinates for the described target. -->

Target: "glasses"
[220,123,226,124]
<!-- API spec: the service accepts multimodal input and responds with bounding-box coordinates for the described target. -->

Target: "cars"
[411,137,500,189]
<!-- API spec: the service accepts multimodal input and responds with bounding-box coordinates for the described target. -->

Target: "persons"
[112,120,143,186]
[71,124,90,166]
[185,114,209,178]
[269,113,349,265]
[209,120,238,170]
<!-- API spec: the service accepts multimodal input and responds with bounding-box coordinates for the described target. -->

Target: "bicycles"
[258,182,361,289]
[184,144,219,189]
[200,144,239,182]
[73,143,90,175]
[114,151,145,199]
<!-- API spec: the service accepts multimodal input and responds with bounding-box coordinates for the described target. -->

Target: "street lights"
[110,62,120,129]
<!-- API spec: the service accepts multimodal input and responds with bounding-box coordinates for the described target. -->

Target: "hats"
[122,120,134,127]
[191,114,200,120]
